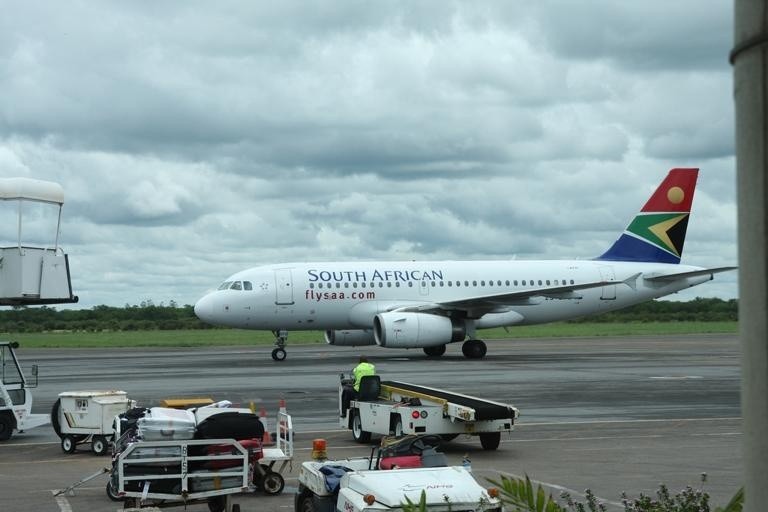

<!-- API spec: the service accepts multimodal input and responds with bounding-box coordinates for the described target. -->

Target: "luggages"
[124,404,264,471]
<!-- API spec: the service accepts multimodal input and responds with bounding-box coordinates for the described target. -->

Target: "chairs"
[356,375,381,402]
[380,434,424,469]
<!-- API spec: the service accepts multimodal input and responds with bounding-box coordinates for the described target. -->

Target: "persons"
[340,355,377,418]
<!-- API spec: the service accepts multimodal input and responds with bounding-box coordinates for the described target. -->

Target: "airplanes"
[194,167,739,359]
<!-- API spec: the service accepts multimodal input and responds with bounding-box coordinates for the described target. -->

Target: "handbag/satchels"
[380,432,425,458]
